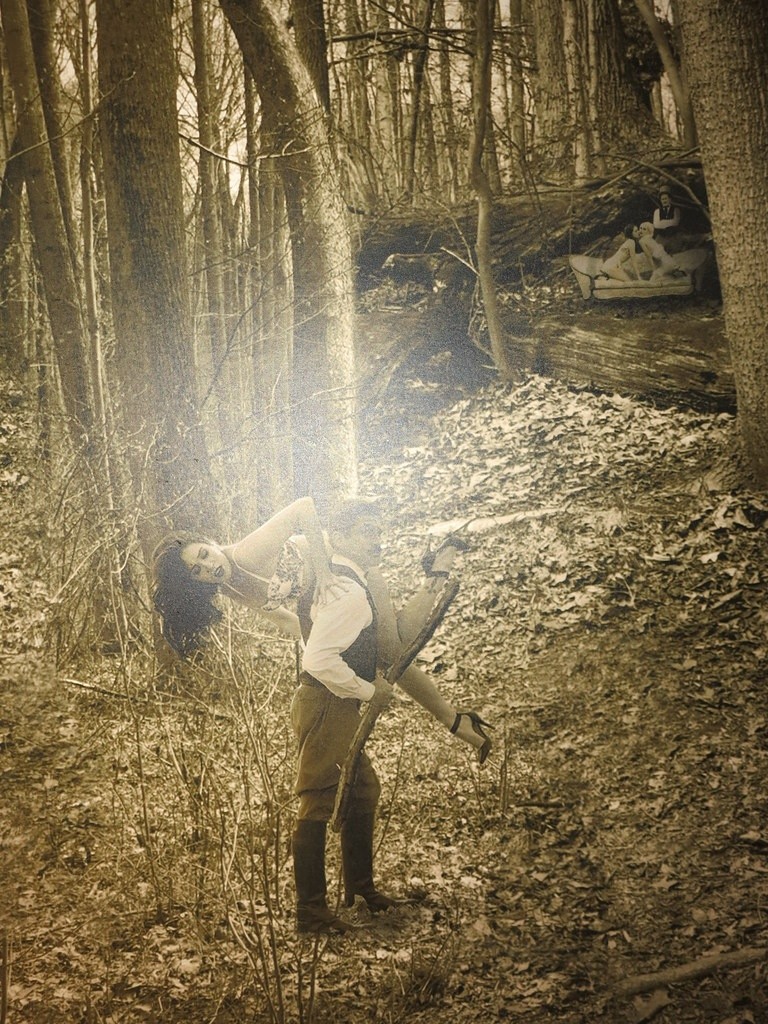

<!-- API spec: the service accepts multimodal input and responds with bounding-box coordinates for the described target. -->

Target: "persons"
[639,221,676,283]
[289,499,408,937]
[653,185,684,254]
[149,495,497,764]
[594,222,643,283]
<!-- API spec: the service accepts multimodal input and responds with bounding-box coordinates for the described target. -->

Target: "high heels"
[420,533,470,579]
[450,710,496,765]
[671,266,687,279]
[593,270,609,281]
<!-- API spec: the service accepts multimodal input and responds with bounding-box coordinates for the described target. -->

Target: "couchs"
[566,248,708,307]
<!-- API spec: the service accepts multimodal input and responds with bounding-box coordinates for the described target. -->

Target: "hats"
[659,185,673,197]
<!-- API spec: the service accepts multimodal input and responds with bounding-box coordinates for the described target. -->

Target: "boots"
[290,819,358,937]
[344,813,420,917]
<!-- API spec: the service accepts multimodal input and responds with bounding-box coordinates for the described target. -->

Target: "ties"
[664,206,667,217]
